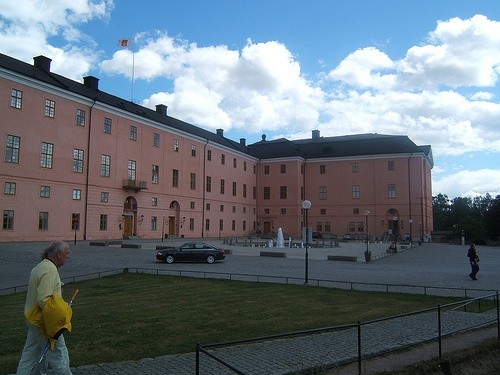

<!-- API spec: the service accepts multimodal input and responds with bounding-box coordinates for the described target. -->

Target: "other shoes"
[469,273,477,280]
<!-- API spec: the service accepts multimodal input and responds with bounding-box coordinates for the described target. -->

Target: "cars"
[156,241,226,264]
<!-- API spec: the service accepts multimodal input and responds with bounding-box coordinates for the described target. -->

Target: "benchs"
[121,244,142,248]
[89,241,109,247]
[327,255,357,262]
[260,251,286,258]
[225,249,232,253]
[156,245,176,250]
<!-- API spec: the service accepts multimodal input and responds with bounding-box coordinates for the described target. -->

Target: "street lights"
[392,216,397,241]
[363,210,371,254]
[419,221,422,239]
[301,200,312,284]
[409,220,412,242]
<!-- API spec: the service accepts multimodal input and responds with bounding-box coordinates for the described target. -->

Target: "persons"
[467,243,480,280]
[16,240,73,375]
[34,248,49,375]
[389,242,395,249]
